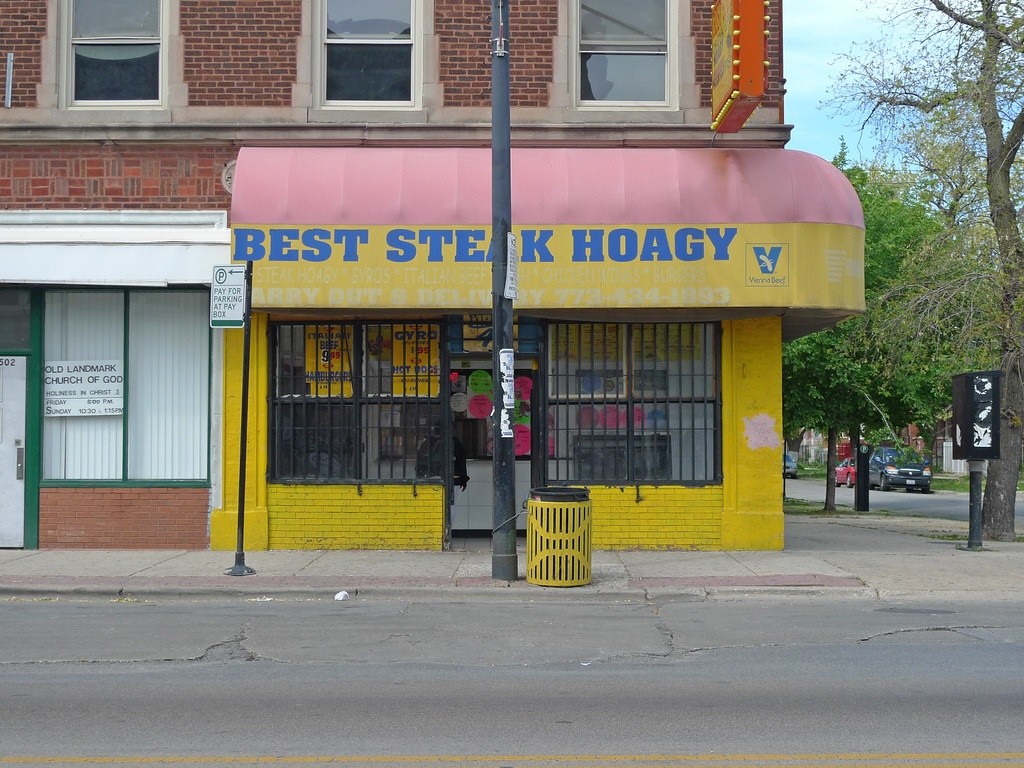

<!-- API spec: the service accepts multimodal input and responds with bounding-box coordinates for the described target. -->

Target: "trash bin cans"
[526,487,593,588]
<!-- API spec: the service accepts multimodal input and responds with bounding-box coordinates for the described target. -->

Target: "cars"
[782,454,798,479]
[869,447,933,494]
[835,457,856,488]
